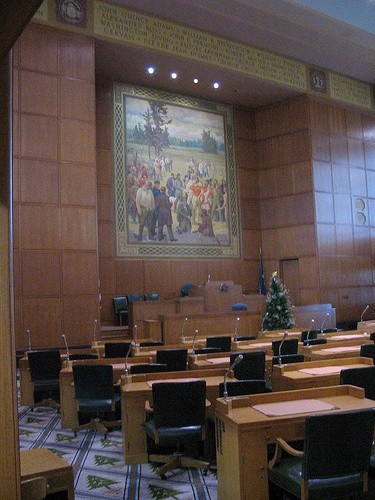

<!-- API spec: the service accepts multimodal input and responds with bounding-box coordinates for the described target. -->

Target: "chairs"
[323,328,337,333]
[105,342,134,359]
[218,379,267,399]
[69,354,99,360]
[145,379,211,480]
[113,297,129,327]
[72,363,122,425]
[229,350,266,380]
[27,351,64,404]
[194,348,221,355]
[300,329,321,342]
[272,354,305,373]
[156,348,189,373]
[340,366,375,401]
[359,343,375,364]
[272,338,299,356]
[129,363,167,375]
[267,407,375,500]
[139,341,165,346]
[206,336,231,352]
[303,339,327,345]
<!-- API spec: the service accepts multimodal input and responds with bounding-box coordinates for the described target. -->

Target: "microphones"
[27,312,330,376]
[360,305,368,322]
[223,355,243,399]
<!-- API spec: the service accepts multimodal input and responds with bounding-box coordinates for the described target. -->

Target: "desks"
[21,281,373,500]
